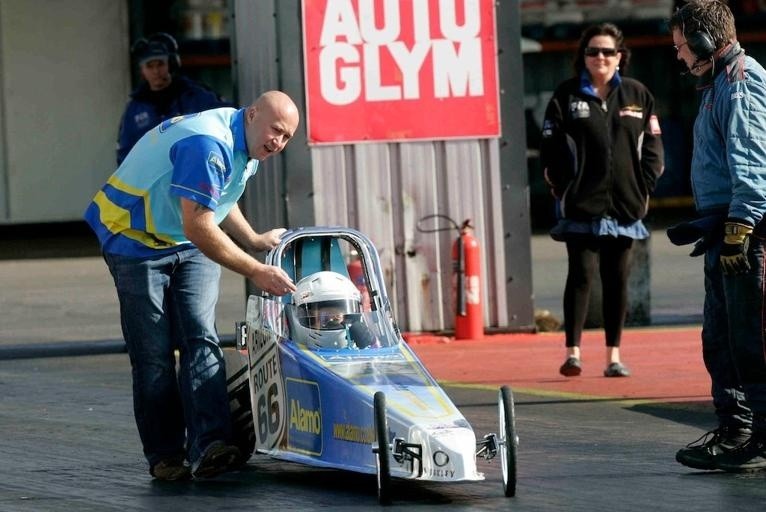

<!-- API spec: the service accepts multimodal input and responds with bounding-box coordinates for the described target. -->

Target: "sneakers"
[676,425,766,473]
[152,456,191,485]
[195,445,240,479]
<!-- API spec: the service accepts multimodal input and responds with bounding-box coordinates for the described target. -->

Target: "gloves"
[719,217,755,275]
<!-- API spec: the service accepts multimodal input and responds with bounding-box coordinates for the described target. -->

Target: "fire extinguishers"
[416,213,485,341]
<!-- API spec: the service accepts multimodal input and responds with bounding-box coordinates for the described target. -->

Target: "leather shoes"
[604,362,629,377]
[560,358,582,376]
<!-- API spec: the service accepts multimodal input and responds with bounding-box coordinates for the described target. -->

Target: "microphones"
[679,63,700,76]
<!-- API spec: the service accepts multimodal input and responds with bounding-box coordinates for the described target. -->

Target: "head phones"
[680,6,717,60]
[148,30,183,74]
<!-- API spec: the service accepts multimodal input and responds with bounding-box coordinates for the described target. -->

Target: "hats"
[137,42,169,66]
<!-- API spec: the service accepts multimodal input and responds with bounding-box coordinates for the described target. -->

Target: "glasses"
[584,46,620,57]
[673,43,685,50]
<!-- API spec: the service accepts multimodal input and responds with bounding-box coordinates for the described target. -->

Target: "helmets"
[290,271,364,350]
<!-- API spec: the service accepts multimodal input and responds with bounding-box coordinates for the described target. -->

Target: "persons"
[84,90,300,485]
[669,0,766,474]
[539,24,666,376]
[117,34,236,168]
[311,302,380,348]
